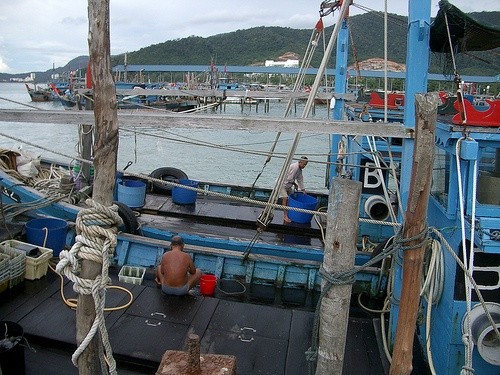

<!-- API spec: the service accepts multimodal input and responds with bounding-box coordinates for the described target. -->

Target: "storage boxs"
[117,265,147,286]
[0,240,53,291]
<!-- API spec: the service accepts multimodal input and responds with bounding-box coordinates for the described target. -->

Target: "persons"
[279,156,308,222]
[155,235,202,296]
[305,84,311,92]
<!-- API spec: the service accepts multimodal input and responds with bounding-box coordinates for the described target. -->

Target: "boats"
[0,0,500,375]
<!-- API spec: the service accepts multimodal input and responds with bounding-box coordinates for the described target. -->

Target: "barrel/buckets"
[200,275,217,295]
[286,193,318,224]
[0,321,25,375]
[117,180,147,208]
[172,179,198,205]
[25,218,68,256]
